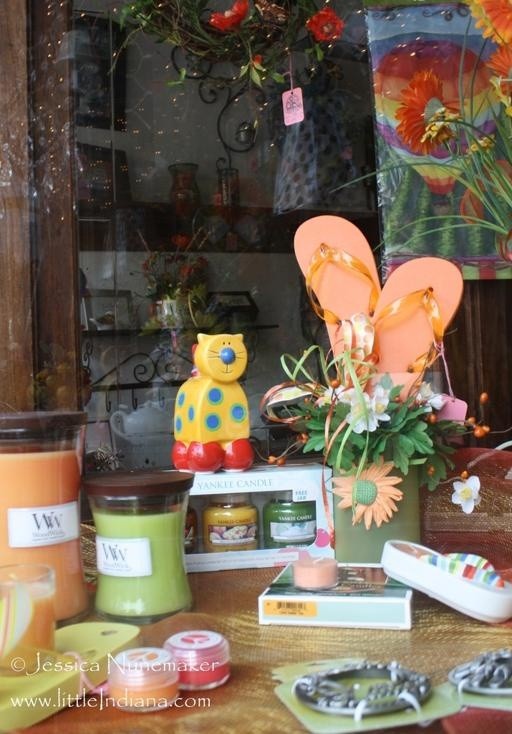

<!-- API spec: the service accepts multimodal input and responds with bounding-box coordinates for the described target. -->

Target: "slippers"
[294,215,463,407]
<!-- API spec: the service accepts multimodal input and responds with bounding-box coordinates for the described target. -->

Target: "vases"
[331,464,422,564]
[156,300,191,331]
[167,161,202,206]
[272,92,344,216]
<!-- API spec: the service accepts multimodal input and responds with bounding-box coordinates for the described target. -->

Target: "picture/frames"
[80,288,136,330]
[75,141,132,205]
[72,9,125,130]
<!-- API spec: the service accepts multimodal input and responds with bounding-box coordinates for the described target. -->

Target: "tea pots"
[110,401,173,441]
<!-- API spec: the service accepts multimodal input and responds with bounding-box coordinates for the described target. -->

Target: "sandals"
[381,537,512,624]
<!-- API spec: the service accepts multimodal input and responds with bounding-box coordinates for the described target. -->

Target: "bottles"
[203,492,315,552]
[1,410,194,624]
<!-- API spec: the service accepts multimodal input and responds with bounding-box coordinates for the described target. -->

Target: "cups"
[1,561,57,679]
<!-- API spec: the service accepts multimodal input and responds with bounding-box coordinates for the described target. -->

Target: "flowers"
[122,215,216,336]
[255,344,490,526]
[105,0,344,144]
[332,0,512,269]
